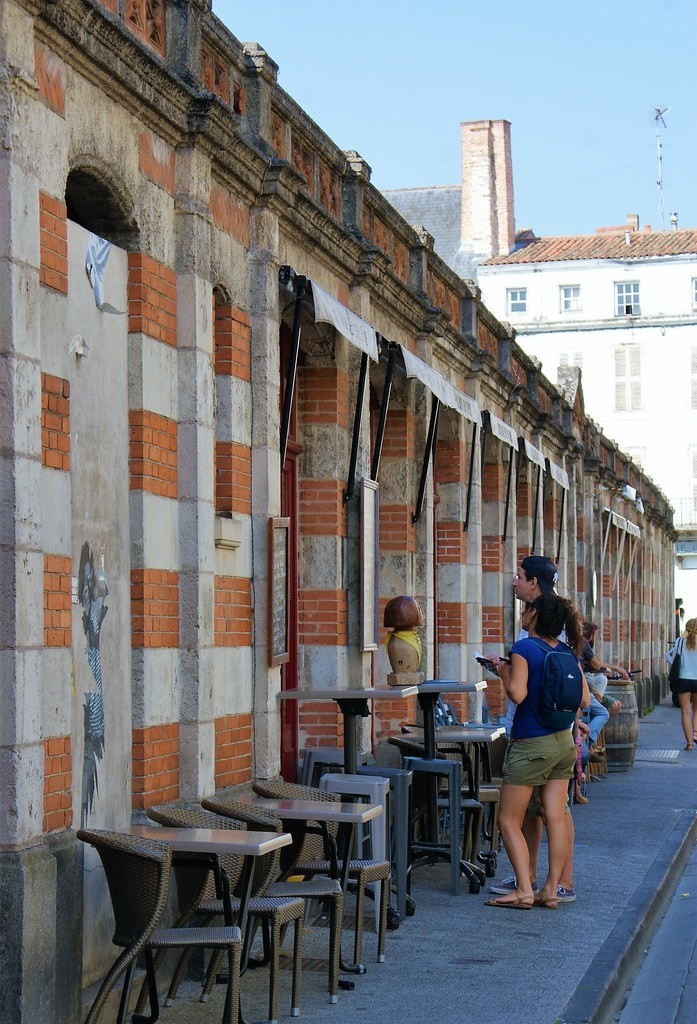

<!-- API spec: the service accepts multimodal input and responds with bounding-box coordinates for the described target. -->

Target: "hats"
[522,556,560,596]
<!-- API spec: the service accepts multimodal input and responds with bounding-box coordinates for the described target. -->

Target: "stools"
[580,707,591,799]
[300,747,415,933]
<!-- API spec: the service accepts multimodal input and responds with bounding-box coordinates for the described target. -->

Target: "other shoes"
[587,744,606,763]
[575,786,588,804]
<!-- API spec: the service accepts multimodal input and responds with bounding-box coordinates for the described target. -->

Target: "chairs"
[75,779,391,1024]
[387,724,512,881]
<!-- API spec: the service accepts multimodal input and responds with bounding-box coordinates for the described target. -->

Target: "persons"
[485,556,577,903]
[482,594,590,910]
[505,622,629,804]
[673,618,697,750]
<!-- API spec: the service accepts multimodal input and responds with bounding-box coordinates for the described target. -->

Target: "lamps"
[599,485,645,513]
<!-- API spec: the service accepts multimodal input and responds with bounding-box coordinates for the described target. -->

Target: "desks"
[224,798,386,990]
[395,680,488,896]
[391,727,505,876]
[117,825,293,1024]
[275,684,423,930]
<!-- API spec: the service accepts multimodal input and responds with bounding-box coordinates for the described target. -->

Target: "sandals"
[692,730,697,744]
[687,744,694,750]
[532,891,558,909]
[484,888,535,909]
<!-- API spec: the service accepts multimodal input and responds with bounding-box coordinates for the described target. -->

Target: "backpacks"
[527,637,584,730]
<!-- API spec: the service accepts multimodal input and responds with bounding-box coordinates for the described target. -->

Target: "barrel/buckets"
[602,684,639,771]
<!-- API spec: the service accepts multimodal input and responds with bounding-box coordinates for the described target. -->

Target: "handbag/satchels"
[668,636,684,682]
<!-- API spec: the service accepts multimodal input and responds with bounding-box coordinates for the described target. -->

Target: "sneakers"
[556,884,577,902]
[488,877,539,895]
